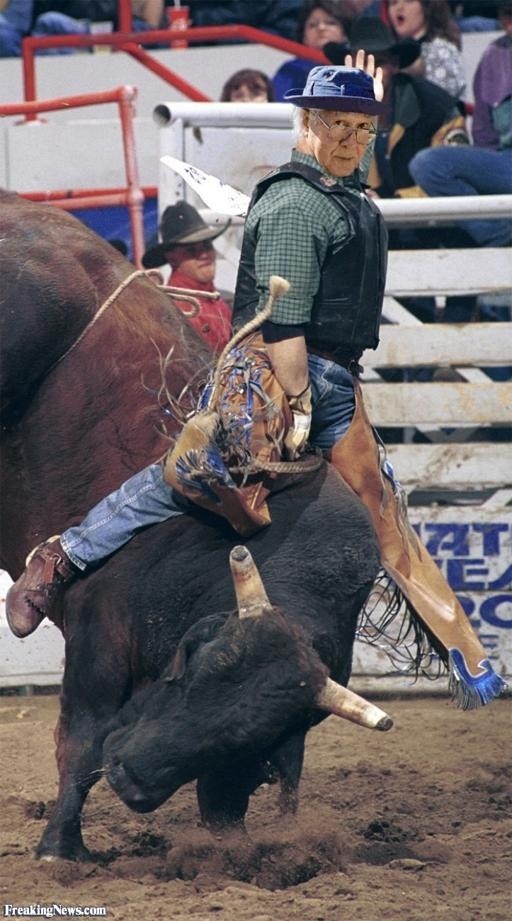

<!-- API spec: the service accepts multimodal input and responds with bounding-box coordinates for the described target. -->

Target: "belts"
[277,385,318,465]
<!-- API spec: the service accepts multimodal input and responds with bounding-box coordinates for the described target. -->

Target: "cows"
[0,183,483,887]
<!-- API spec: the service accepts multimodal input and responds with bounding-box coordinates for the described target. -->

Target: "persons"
[319,9,468,448]
[266,0,358,104]
[377,0,473,101]
[134,191,237,375]
[0,47,511,715]
[406,1,511,324]
[217,67,276,103]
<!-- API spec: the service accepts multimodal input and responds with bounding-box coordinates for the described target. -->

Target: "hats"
[319,15,426,70]
[283,65,390,117]
[139,198,233,270]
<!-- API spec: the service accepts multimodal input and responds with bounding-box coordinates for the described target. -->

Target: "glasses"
[310,110,378,148]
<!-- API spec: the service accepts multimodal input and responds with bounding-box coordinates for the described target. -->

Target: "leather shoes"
[5,534,82,640]
